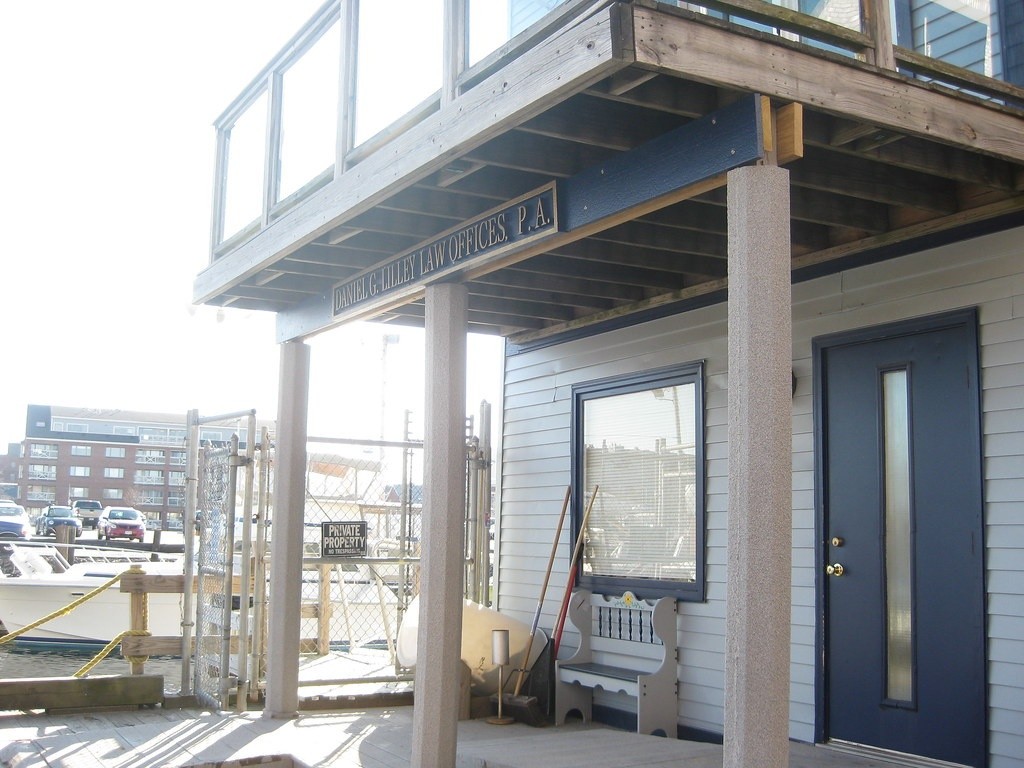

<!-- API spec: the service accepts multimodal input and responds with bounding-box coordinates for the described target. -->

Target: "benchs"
[555,590,678,739]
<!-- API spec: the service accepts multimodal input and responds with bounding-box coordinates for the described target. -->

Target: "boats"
[1,546,407,657]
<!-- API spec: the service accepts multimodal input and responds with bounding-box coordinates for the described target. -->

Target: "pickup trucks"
[71,500,104,527]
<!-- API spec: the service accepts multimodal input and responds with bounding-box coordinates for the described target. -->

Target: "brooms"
[488,485,571,728]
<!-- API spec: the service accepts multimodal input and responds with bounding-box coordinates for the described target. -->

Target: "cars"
[35,504,83,537]
[196,511,214,533]
[97,507,146,542]
[0,498,33,540]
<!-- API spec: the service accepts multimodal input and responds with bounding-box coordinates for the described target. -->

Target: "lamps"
[487,631,514,725]
